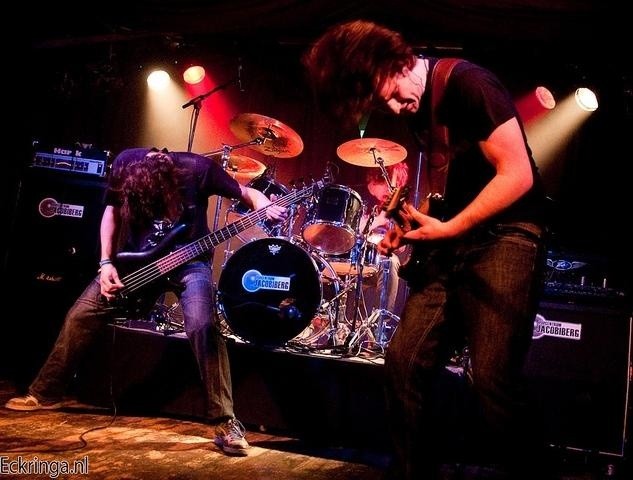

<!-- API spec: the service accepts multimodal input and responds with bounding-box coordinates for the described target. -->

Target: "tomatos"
[540,282,627,314]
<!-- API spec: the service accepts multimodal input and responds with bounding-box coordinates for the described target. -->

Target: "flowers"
[239,63,247,91]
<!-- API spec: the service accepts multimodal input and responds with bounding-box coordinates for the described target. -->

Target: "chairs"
[214,417,249,454]
[5,392,64,411]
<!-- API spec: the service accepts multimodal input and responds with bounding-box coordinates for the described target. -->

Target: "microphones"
[239,63,247,91]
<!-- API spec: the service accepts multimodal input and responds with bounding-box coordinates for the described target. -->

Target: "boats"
[526,277,625,448]
[5,166,108,266]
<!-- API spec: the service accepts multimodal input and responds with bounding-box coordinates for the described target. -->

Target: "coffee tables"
[220,155,266,175]
[336,138,408,167]
[230,113,304,159]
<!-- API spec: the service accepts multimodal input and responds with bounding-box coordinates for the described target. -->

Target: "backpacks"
[218,233,341,347]
[224,178,300,244]
[300,182,415,291]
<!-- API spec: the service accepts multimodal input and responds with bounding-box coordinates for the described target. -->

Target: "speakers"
[526,277,625,448]
[5,166,108,266]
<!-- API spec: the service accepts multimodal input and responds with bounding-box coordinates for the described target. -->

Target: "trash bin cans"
[99,259,112,267]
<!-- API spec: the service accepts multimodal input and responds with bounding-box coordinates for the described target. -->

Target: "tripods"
[165,151,232,320]
[333,191,393,358]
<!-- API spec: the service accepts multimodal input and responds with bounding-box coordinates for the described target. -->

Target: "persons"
[302,21,547,479]
[3,148,289,455]
[317,161,409,323]
